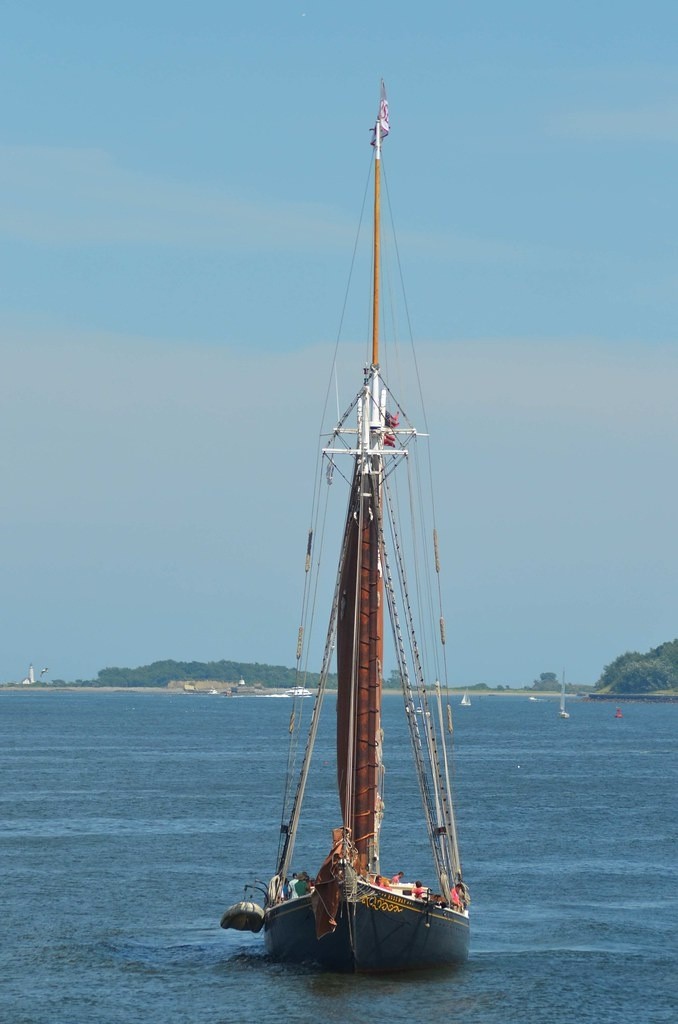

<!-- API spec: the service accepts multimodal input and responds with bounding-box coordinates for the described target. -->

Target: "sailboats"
[219,76,471,977]
[459,686,471,706]
[558,667,569,718]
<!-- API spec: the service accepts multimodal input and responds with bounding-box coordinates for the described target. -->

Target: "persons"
[375,872,464,912]
[281,872,315,901]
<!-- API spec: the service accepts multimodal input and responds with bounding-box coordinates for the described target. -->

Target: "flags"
[371,82,389,146]
[384,411,399,448]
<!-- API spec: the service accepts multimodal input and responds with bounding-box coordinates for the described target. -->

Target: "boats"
[614,707,622,718]
[286,686,313,696]
[208,689,220,695]
[224,690,232,698]
